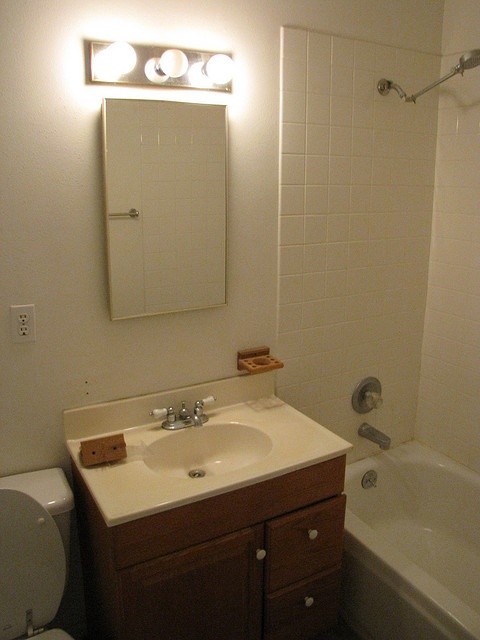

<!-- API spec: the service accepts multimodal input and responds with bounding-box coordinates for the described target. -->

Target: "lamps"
[207,54,235,84]
[105,42,136,74]
[161,49,187,77]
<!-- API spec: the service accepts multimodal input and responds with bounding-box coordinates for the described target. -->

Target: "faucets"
[357,423,392,451]
[153,395,216,431]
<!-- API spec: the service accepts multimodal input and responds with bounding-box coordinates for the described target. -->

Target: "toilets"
[0,468,75,640]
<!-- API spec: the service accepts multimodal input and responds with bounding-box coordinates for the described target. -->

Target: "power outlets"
[9,304,37,343]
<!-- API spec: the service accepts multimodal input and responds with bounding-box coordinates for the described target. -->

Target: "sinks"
[141,423,274,482]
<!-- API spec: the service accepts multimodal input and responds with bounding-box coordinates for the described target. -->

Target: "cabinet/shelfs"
[72,453,347,639]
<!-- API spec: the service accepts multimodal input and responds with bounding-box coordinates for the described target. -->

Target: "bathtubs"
[343,440,479,640]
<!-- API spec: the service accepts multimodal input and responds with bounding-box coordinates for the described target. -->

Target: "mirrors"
[102,98,228,321]
